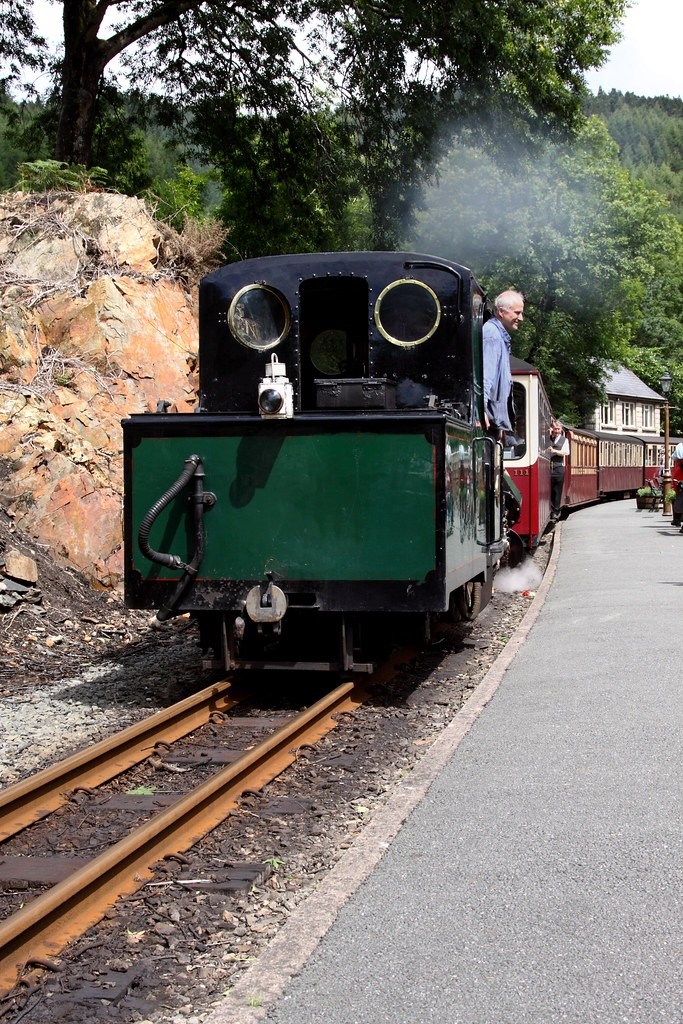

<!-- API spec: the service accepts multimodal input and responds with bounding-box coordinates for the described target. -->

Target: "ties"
[553,437,556,443]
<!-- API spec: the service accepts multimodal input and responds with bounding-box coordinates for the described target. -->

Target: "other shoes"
[670,519,681,528]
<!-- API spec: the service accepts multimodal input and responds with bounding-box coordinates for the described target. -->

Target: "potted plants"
[636,486,662,509]
[664,488,683,522]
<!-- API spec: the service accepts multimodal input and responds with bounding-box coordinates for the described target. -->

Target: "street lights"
[654,371,677,516]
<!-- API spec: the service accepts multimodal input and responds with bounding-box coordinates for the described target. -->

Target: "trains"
[117,249,683,681]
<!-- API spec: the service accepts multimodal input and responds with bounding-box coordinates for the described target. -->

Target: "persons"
[548,420,570,519]
[504,416,525,448]
[483,289,525,553]
[670,442,683,533]
